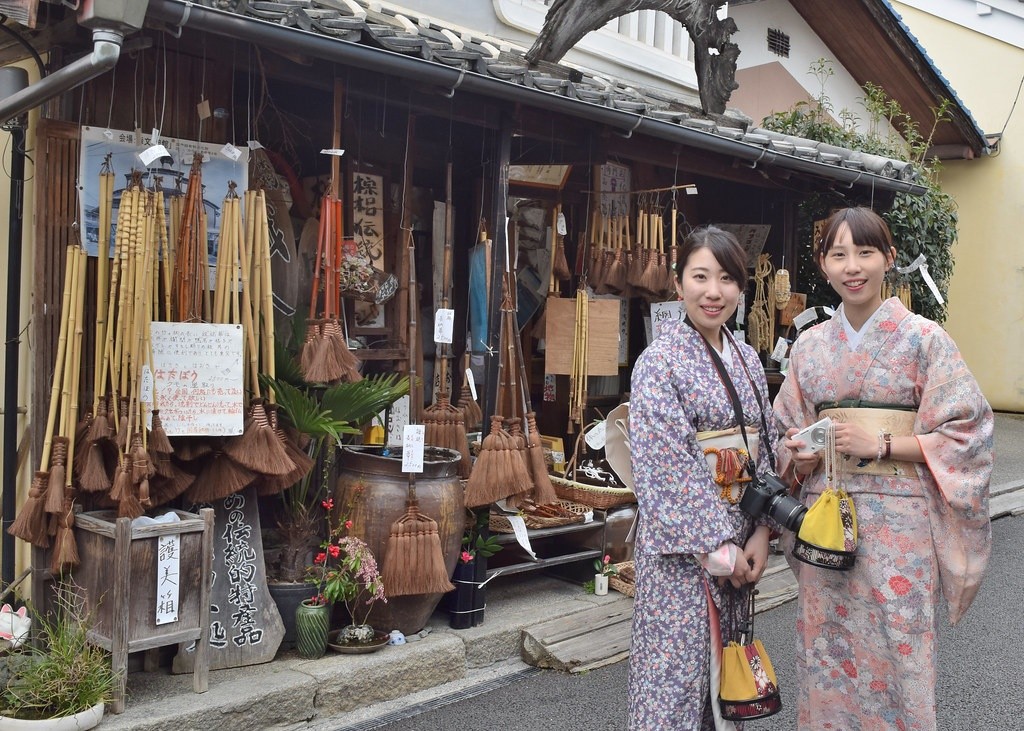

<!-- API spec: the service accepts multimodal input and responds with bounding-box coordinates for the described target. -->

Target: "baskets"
[603,561,636,599]
[548,423,639,510]
[488,498,593,534]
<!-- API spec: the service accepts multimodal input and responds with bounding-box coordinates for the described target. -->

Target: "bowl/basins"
[386,630,406,645]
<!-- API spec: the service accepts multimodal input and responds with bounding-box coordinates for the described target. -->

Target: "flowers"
[323,537,387,626]
[306,433,369,606]
[594,554,619,577]
[460,512,505,562]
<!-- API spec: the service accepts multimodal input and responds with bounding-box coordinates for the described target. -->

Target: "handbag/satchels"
[790,421,856,571]
[719,583,782,722]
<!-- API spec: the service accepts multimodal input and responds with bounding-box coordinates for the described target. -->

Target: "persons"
[772,207,994,731]
[627,228,779,731]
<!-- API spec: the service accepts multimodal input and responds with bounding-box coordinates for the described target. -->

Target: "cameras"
[791,417,833,454]
[740,471,808,532]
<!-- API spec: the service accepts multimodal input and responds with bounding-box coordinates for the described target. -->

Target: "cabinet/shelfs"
[73,499,213,715]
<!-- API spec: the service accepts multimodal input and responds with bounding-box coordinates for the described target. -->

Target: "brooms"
[380,245,457,597]
[529,206,571,338]
[301,194,363,385]
[587,208,677,294]
[421,245,482,477]
[461,307,558,508]
[6,153,315,574]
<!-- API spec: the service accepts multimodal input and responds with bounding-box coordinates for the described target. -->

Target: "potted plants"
[0,557,133,731]
[594,574,608,596]
[266,322,423,649]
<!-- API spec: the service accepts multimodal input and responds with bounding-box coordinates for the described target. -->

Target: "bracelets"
[883,432,893,461]
[874,433,882,463]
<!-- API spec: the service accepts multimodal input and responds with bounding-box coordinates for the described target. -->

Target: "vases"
[336,623,375,646]
[295,599,329,659]
[451,560,474,630]
